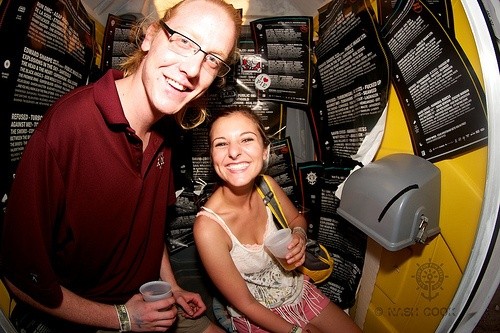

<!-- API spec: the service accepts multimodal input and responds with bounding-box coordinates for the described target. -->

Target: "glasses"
[159,19,232,78]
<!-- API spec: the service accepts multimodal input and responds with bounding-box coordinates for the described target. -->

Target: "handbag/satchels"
[253,173,334,288]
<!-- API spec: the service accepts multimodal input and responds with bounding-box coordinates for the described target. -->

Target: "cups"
[139,281,174,311]
[264,228,301,271]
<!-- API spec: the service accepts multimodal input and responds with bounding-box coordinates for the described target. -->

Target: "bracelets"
[293,226,307,240]
[287,324,303,333]
[114,304,131,332]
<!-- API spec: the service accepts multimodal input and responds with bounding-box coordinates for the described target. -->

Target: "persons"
[0,0,245,333]
[191,106,365,333]
